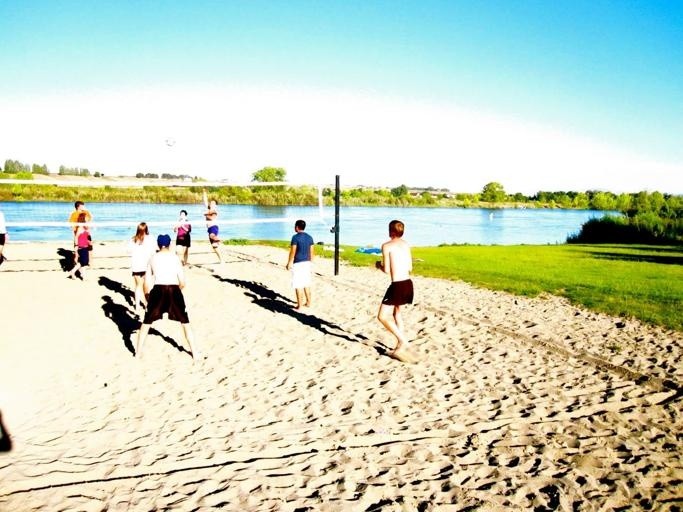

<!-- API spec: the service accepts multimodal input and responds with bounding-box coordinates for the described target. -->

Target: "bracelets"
[73,244,78,247]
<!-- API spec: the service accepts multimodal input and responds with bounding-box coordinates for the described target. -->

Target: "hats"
[158,235,171,248]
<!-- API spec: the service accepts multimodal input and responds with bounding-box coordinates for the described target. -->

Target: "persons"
[125,222,156,312]
[133,234,201,360]
[0,211,7,265]
[173,210,193,266]
[285,219,315,310]
[376,219,415,364]
[67,213,92,281]
[201,188,225,263]
[67,201,95,267]
